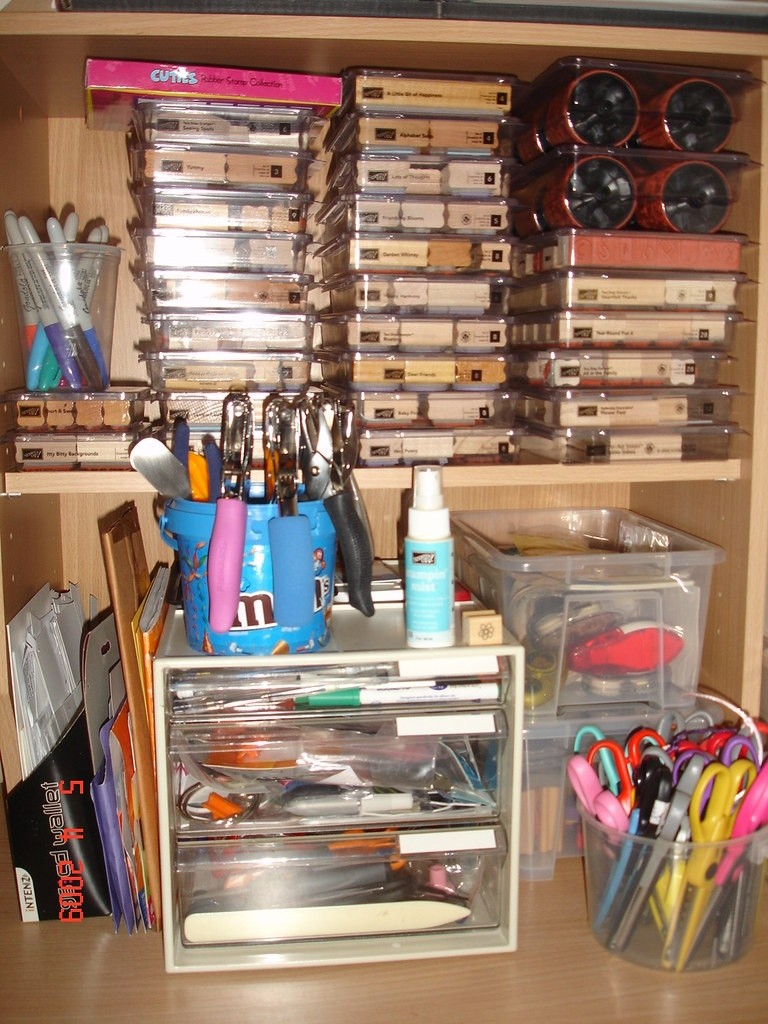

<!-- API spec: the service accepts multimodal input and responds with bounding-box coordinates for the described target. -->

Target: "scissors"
[565,717,768,972]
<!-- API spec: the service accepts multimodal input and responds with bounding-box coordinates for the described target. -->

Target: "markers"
[294,682,504,707]
[4,205,112,395]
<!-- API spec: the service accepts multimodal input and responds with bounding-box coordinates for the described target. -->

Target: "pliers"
[168,384,379,632]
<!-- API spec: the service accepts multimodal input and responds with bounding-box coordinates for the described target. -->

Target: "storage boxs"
[496,693,737,883]
[82,59,343,134]
[308,65,535,467]
[0,386,149,463]
[125,99,331,460]
[450,506,727,719]
[508,227,762,466]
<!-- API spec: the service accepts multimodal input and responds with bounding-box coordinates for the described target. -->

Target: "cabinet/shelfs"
[0,12,767,722]
[154,577,525,974]
